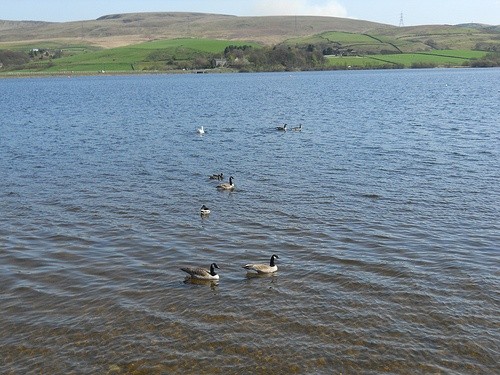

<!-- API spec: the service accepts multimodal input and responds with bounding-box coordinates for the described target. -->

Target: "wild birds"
[209,172,223,180]
[198,126,205,135]
[276,123,287,131]
[179,262,220,280]
[215,176,234,189]
[200,204,210,215]
[291,123,302,131]
[242,254,280,273]
